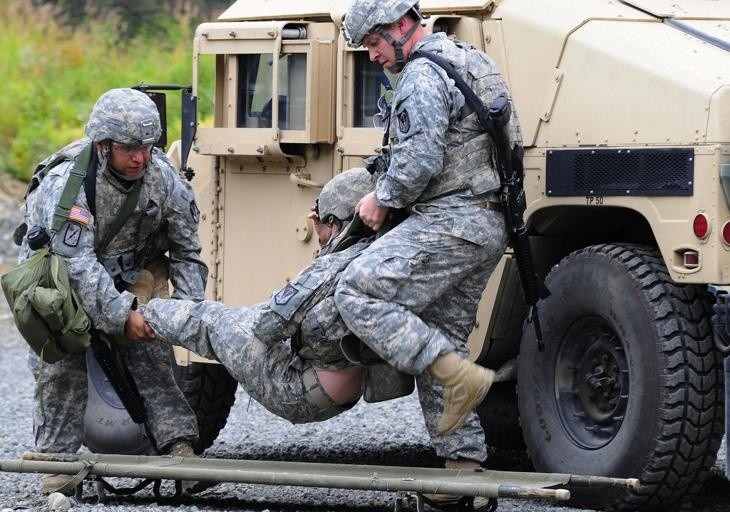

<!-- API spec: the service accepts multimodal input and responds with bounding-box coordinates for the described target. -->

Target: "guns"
[487,92,550,351]
[25,225,156,452]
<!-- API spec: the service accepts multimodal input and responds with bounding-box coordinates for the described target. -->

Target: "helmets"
[83,82,165,150]
[317,167,381,223]
[341,0,432,51]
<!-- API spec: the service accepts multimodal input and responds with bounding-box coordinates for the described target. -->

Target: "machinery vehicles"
[81,1,729,507]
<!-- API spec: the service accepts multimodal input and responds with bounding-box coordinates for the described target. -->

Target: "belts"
[303,374,339,418]
[475,201,503,212]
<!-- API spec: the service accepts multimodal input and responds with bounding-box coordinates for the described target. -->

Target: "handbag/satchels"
[2,160,147,364]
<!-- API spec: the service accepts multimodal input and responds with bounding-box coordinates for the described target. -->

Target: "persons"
[333,0,526,512]
[17,87,225,497]
[124,167,384,425]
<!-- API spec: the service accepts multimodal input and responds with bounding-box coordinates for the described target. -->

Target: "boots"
[165,440,232,495]
[36,466,79,497]
[427,353,497,437]
[149,250,174,305]
[126,267,156,309]
[422,455,490,504]
